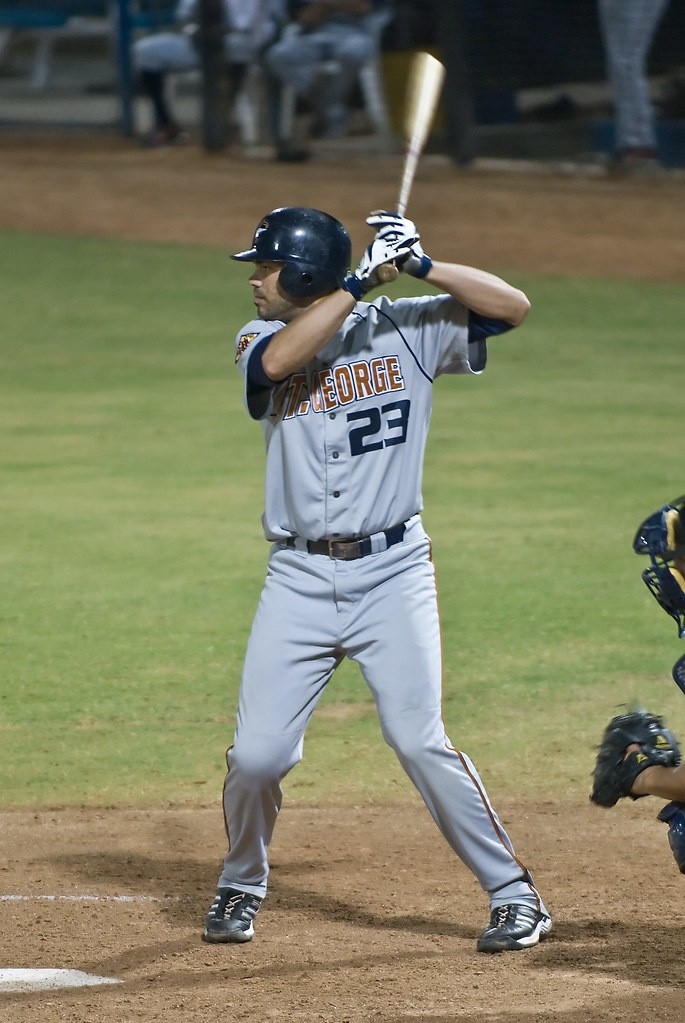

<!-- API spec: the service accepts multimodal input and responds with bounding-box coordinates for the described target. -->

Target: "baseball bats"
[379,50,447,283]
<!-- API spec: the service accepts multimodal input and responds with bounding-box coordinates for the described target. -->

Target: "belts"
[286,523,407,561]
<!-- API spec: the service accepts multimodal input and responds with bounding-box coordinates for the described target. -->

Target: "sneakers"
[204,886,262,942]
[476,904,552,954]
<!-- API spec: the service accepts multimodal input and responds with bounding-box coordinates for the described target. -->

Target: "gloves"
[338,209,431,302]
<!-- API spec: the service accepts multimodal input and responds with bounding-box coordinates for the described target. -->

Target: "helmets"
[228,205,351,298]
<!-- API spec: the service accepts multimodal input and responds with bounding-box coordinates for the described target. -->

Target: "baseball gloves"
[586,701,681,811]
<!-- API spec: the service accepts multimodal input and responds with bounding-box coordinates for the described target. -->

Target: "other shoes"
[608,145,658,178]
[142,124,180,144]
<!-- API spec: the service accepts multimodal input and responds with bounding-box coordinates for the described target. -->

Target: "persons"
[132,0,272,149]
[590,497,685,873]
[202,205,553,952]
[268,0,384,141]
